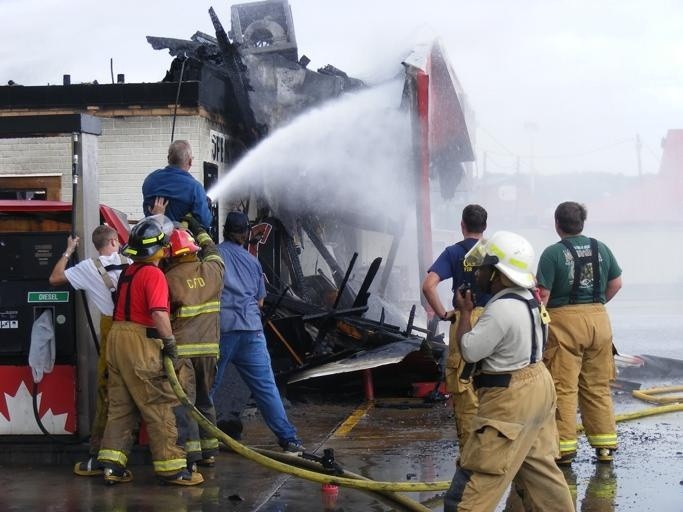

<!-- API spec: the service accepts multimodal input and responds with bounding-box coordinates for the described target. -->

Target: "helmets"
[462,231,538,289]
[120,214,174,260]
[167,229,200,258]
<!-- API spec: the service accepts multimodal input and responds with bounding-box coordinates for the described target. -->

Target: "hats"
[224,212,247,234]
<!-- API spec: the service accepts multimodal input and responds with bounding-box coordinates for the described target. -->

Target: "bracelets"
[62,252,69,258]
[440,312,447,320]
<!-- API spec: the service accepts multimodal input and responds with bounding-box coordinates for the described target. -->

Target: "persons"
[141,139,213,256]
[159,214,225,469]
[536,201,622,464]
[93,220,205,486]
[48,197,168,476]
[210,211,315,458]
[422,204,486,464]
[440,230,576,512]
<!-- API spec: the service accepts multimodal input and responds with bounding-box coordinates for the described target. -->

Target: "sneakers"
[72,455,215,485]
[596,448,613,461]
[554,450,577,465]
[282,442,306,457]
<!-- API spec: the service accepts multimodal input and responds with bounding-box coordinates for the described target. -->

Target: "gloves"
[163,335,177,369]
